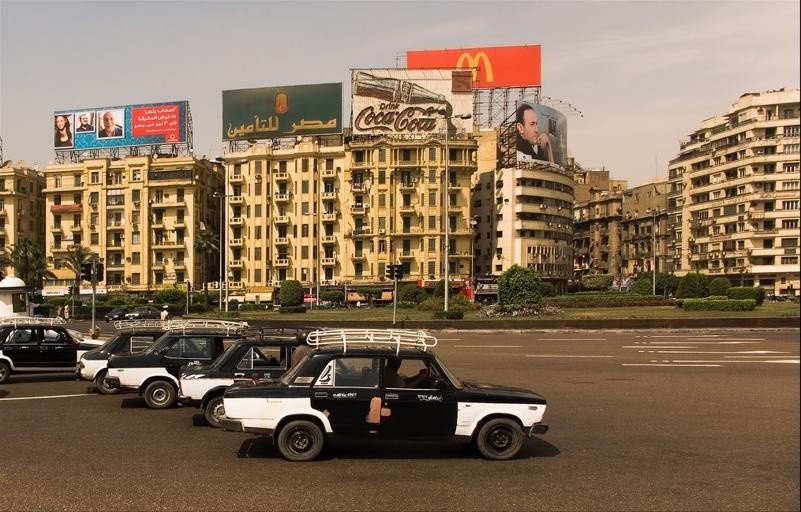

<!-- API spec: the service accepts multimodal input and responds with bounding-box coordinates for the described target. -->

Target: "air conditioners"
[120,234,125,238]
[413,178,418,182]
[256,174,261,179]
[539,203,571,230]
[380,229,385,233]
[336,211,341,215]
[287,190,293,194]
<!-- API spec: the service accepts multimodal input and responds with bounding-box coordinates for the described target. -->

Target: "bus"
[301,285,346,304]
[345,280,418,303]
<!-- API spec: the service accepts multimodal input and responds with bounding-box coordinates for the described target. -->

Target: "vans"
[613,273,637,292]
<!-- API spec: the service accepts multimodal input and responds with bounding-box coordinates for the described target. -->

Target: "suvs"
[177,325,351,429]
[107,320,279,409]
[0,317,106,382]
[78,318,248,393]
[224,328,550,460]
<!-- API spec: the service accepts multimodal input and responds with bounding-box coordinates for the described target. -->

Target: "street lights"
[212,191,224,311]
[214,155,231,313]
[645,207,662,295]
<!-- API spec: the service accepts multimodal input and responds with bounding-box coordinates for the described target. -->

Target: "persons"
[516,105,554,164]
[76,114,93,131]
[386,359,422,387]
[99,112,122,136]
[55,116,72,147]
[161,305,169,320]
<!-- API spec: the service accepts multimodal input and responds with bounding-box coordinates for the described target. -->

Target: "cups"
[436,116,464,141]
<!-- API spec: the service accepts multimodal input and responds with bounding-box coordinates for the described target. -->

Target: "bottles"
[355,71,447,105]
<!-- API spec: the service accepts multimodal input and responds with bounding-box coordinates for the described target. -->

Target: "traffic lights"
[394,264,400,279]
[386,265,391,278]
[80,264,87,280]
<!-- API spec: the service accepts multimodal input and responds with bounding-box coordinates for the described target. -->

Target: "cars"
[768,294,795,300]
[124,307,162,320]
[105,305,134,321]
